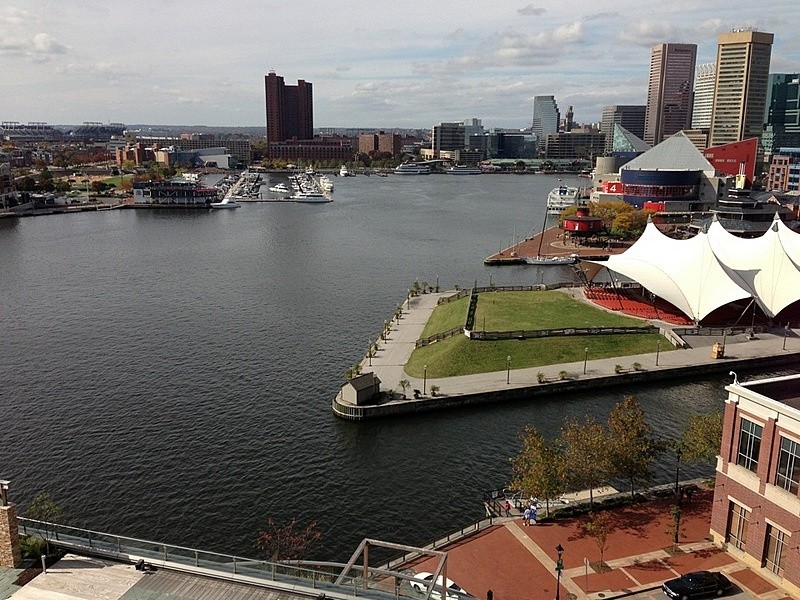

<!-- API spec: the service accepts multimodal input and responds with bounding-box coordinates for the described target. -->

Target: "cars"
[410,571,467,600]
[83,198,104,204]
[64,199,81,204]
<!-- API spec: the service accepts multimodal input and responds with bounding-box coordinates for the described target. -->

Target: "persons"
[505,495,538,526]
[395,569,403,595]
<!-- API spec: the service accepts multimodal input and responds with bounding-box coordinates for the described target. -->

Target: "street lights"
[507,355,511,384]
[723,331,726,355]
[541,271,544,284]
[415,276,418,296]
[674,486,684,541]
[555,544,565,600]
[350,288,410,379]
[656,339,660,366]
[783,322,788,349]
[437,274,439,291]
[424,364,427,394]
[584,347,589,374]
[675,449,682,493]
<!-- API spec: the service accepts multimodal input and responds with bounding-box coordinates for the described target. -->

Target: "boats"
[320,177,334,192]
[546,184,580,215]
[443,166,481,174]
[210,199,241,209]
[339,165,349,177]
[393,160,430,175]
[232,170,326,200]
